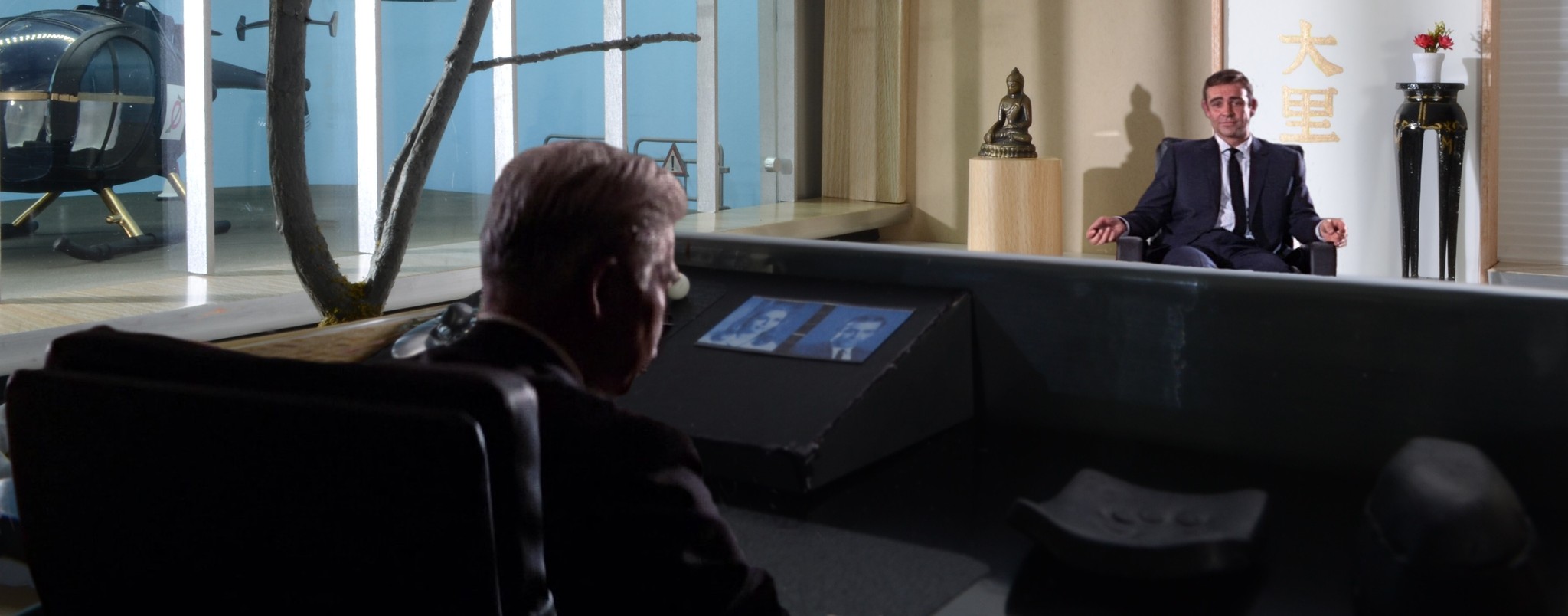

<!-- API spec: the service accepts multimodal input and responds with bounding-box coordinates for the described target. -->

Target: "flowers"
[1413,24,1454,51]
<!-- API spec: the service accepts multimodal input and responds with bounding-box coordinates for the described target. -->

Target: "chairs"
[1115,136,1338,275]
[6,321,553,616]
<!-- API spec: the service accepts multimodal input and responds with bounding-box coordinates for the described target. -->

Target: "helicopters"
[0,0,338,261]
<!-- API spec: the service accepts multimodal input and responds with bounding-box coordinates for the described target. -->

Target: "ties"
[832,349,845,361]
[1226,148,1247,254]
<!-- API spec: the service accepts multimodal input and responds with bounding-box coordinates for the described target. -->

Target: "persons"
[984,67,1032,143]
[701,304,790,350]
[793,314,886,362]
[1089,70,1348,271]
[363,139,787,615]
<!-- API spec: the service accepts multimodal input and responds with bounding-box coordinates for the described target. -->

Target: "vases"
[1423,52,1445,82]
